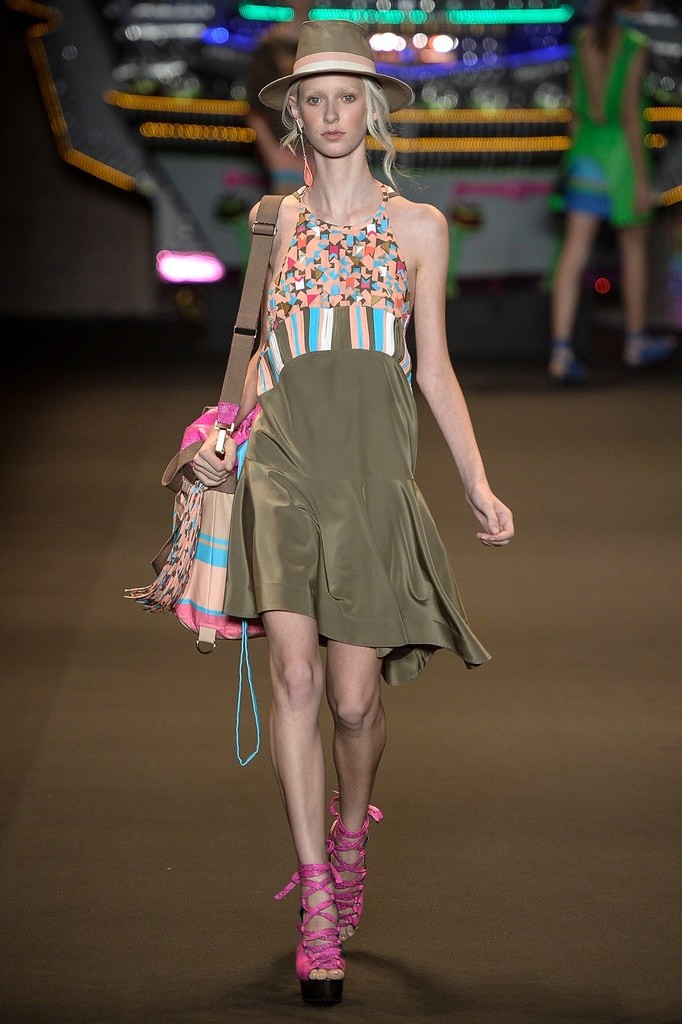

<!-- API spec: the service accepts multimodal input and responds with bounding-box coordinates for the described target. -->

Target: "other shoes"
[626,336,672,367]
[547,343,578,376]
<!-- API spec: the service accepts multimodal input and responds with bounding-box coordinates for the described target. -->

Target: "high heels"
[329,789,384,942]
[273,865,345,1007]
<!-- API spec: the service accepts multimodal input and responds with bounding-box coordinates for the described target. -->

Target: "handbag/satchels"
[123,407,266,654]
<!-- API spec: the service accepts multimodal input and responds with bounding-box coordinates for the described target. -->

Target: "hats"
[258,20,415,114]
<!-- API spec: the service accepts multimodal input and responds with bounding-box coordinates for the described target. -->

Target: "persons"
[193,18,514,996]
[542,1,669,389]
